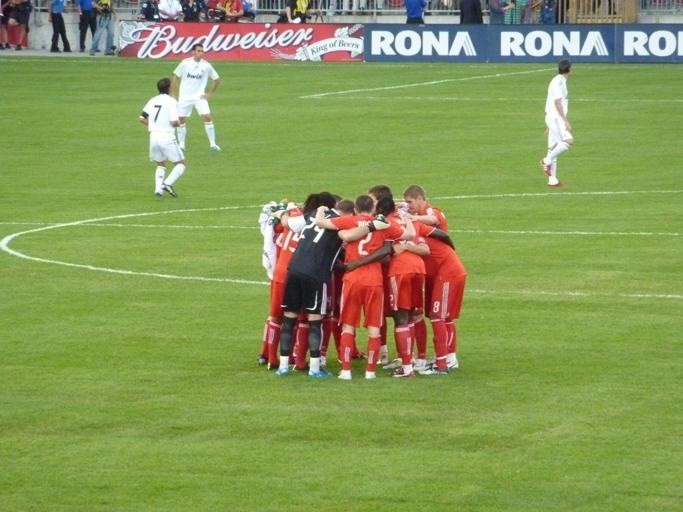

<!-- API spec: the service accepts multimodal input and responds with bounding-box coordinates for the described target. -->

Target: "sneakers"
[209,144,221,152]
[540,158,552,176]
[161,183,177,198]
[258,352,459,381]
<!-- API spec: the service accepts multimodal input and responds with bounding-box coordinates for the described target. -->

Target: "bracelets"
[367,221,377,233]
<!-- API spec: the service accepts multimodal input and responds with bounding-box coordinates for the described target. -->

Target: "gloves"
[269,204,288,218]
[367,214,390,231]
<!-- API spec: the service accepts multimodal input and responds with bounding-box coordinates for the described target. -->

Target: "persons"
[170,44,224,152]
[136,0,312,24]
[540,60,574,187]
[0,1,33,51]
[258,187,467,381]
[405,0,556,24]
[47,0,73,52]
[75,0,100,52]
[89,0,118,55]
[137,78,187,197]
